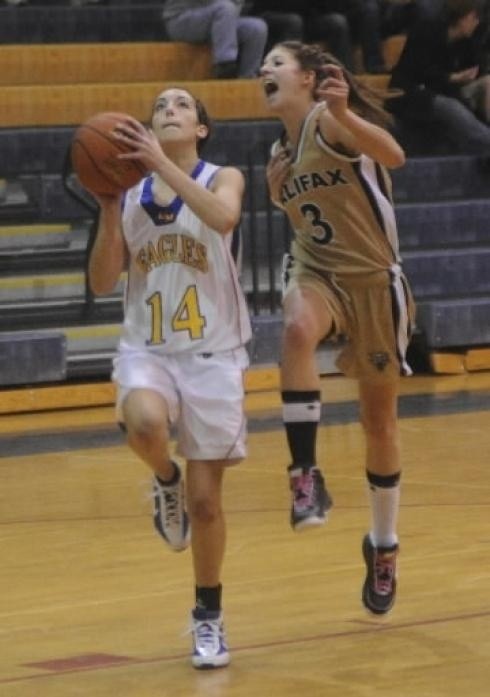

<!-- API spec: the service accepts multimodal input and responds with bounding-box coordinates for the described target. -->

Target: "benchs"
[0,0,490,385]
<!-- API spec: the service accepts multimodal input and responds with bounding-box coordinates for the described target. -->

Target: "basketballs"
[71,112,149,197]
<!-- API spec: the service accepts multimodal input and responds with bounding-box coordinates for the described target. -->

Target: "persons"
[259,34,422,620]
[162,0,489,151]
[83,83,254,670]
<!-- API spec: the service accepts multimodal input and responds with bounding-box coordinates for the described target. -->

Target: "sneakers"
[356,533,402,617]
[287,458,333,533]
[187,611,231,671]
[147,459,191,551]
